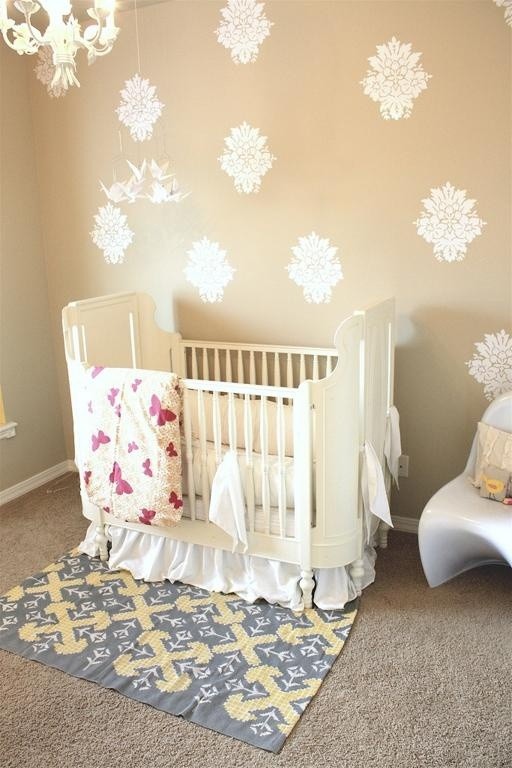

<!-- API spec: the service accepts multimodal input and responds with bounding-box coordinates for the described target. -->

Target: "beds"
[61,290,396,610]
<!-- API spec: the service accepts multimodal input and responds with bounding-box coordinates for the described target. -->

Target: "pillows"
[468,421,511,503]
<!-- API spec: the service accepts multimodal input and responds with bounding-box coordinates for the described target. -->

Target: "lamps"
[1,0,122,91]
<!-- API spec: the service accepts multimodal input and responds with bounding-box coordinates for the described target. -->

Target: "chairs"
[417,392,512,588]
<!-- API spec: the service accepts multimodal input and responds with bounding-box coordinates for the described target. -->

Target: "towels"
[383,405,402,496]
[359,439,395,545]
[0,544,361,754]
[207,449,249,555]
